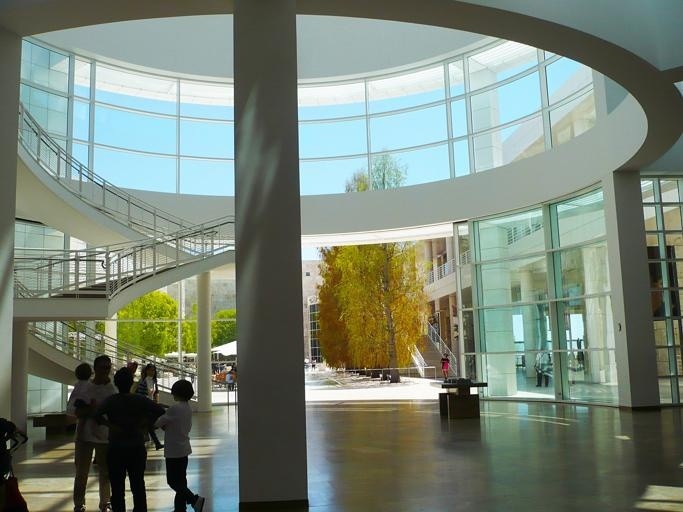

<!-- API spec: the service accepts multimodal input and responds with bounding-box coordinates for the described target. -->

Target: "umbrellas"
[210,340,237,356]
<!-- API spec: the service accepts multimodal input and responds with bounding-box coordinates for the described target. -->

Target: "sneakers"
[75,505,85,511]
[156,444,164,450]
[102,509,111,512]
[193,496,204,512]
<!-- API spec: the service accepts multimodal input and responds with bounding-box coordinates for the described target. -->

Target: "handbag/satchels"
[4,476,29,512]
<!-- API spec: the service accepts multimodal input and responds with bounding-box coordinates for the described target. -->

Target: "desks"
[439,383,487,419]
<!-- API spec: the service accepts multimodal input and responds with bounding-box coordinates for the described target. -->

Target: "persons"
[221,362,236,391]
[536,352,550,387]
[65,356,166,512]
[312,358,316,367]
[440,353,450,379]
[0,418,25,512]
[155,379,205,512]
[304,356,309,369]
